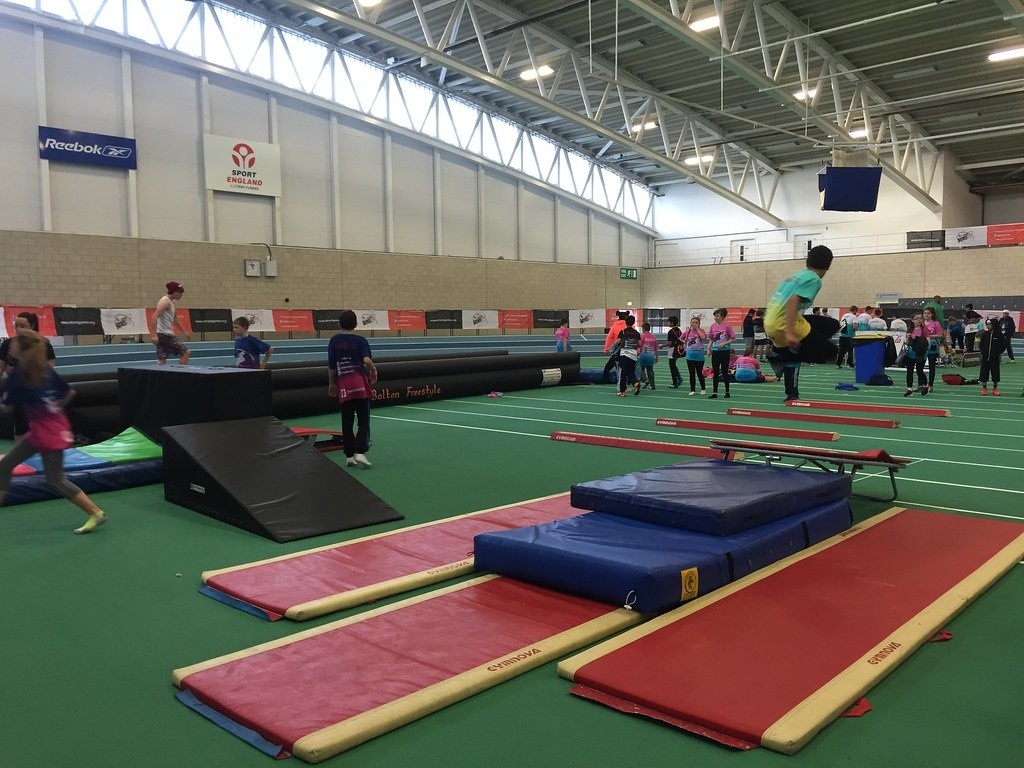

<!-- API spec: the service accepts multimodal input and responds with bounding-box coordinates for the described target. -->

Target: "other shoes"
[913,387,921,393]
[708,394,719,399]
[921,385,931,395]
[1009,359,1016,363]
[723,393,731,398]
[981,388,988,396]
[791,397,797,401]
[617,392,627,397]
[649,385,655,390]
[700,390,707,395]
[642,381,649,390]
[783,396,791,402]
[689,390,697,396]
[929,387,933,393]
[633,383,640,395]
[667,384,679,389]
[903,390,914,397]
[992,388,1000,396]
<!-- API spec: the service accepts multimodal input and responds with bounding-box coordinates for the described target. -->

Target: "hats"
[166,281,184,294]
[1002,309,1012,314]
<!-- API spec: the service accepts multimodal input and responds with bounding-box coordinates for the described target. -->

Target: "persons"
[979,319,1009,395]
[923,295,951,352]
[811,307,831,318]
[604,312,684,397]
[947,303,985,354]
[904,311,931,397]
[707,308,736,398]
[678,317,707,396]
[233,317,274,369]
[998,309,1016,364]
[717,348,777,383]
[151,282,191,365]
[0,312,109,534]
[763,245,840,402]
[743,308,774,364]
[555,319,571,352]
[836,305,907,368]
[912,307,944,392]
[329,310,377,466]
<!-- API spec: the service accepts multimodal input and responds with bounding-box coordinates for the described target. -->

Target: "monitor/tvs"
[818,166,883,212]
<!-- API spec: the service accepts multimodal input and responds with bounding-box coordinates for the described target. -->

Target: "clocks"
[245,259,262,277]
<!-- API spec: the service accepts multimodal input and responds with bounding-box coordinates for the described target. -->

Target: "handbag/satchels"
[896,342,909,368]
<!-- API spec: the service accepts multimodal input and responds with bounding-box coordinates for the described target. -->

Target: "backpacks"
[671,329,688,359]
